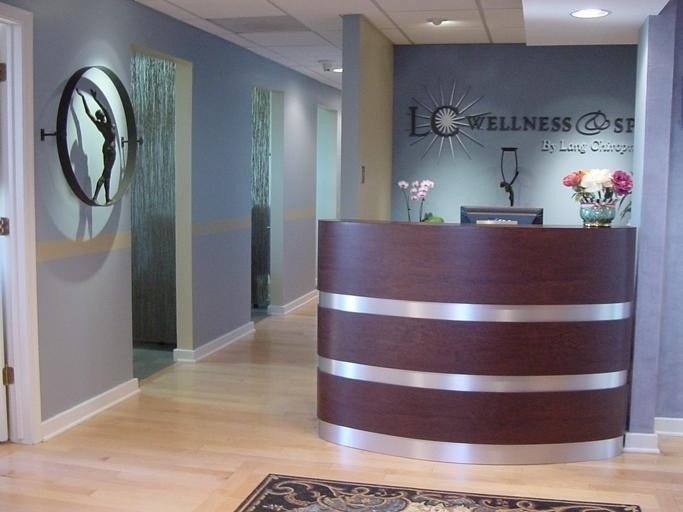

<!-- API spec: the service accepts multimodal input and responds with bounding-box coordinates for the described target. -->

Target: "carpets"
[232,471,641,512]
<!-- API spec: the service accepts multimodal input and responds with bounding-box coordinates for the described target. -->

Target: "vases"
[578,201,616,226]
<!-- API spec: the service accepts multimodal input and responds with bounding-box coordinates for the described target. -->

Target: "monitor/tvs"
[460,205,543,225]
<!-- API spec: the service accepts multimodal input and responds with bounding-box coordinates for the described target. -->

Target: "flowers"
[396,178,445,224]
[562,169,633,208]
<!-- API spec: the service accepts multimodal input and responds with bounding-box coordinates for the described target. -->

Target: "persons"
[75,87,115,206]
